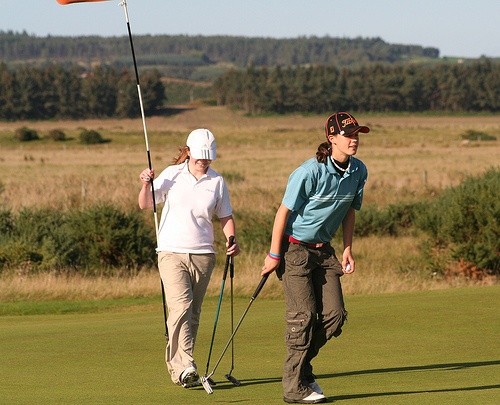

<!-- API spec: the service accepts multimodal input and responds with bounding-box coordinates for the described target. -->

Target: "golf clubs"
[199,271,271,395]
[224,255,240,386]
[204,235,235,385]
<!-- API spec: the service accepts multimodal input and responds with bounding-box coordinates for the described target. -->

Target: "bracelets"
[268,250,281,261]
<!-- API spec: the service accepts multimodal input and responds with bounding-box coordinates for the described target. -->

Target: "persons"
[138,128,239,388]
[260,111,370,405]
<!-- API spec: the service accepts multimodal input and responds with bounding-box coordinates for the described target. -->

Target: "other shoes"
[180,366,199,388]
[285,381,327,404]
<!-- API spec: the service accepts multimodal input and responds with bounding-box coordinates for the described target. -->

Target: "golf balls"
[345,263,351,271]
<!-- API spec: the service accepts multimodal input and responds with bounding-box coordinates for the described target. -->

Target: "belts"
[282,234,330,249]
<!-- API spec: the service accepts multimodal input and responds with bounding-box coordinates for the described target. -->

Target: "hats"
[186,128,217,161]
[325,112,370,139]
